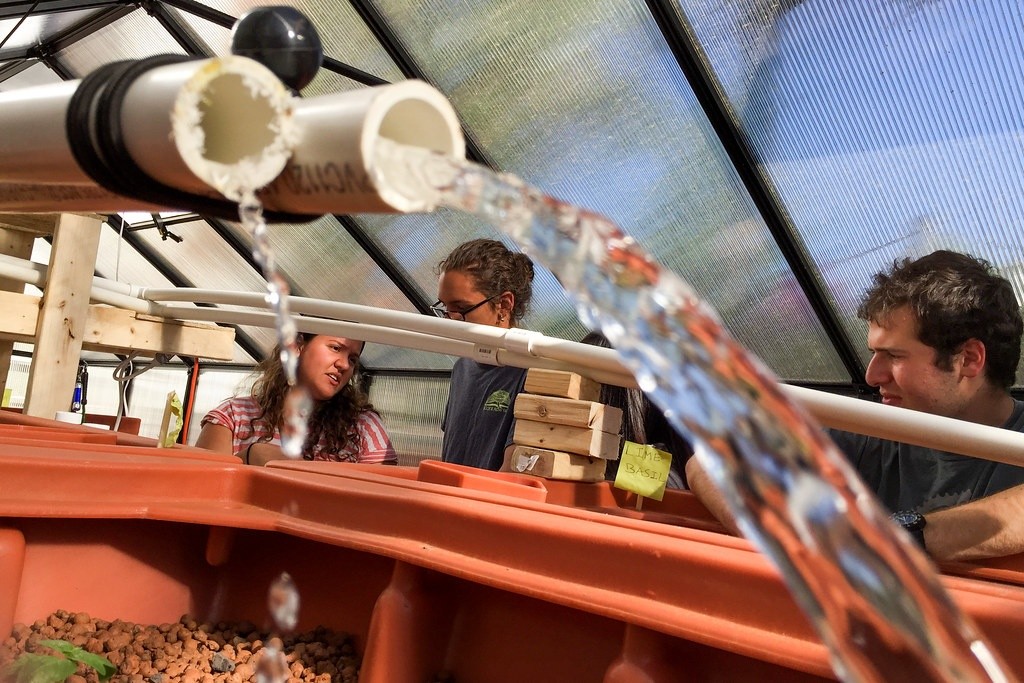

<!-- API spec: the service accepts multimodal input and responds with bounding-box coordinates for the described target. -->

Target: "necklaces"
[284,402,312,417]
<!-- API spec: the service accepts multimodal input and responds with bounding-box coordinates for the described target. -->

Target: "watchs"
[889,510,926,552]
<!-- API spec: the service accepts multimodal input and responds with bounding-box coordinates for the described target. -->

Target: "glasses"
[429,292,503,321]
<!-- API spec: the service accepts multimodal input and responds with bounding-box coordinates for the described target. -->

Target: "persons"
[437,239,535,473]
[685,251,1024,562]
[195,315,399,467]
[581,332,693,492]
